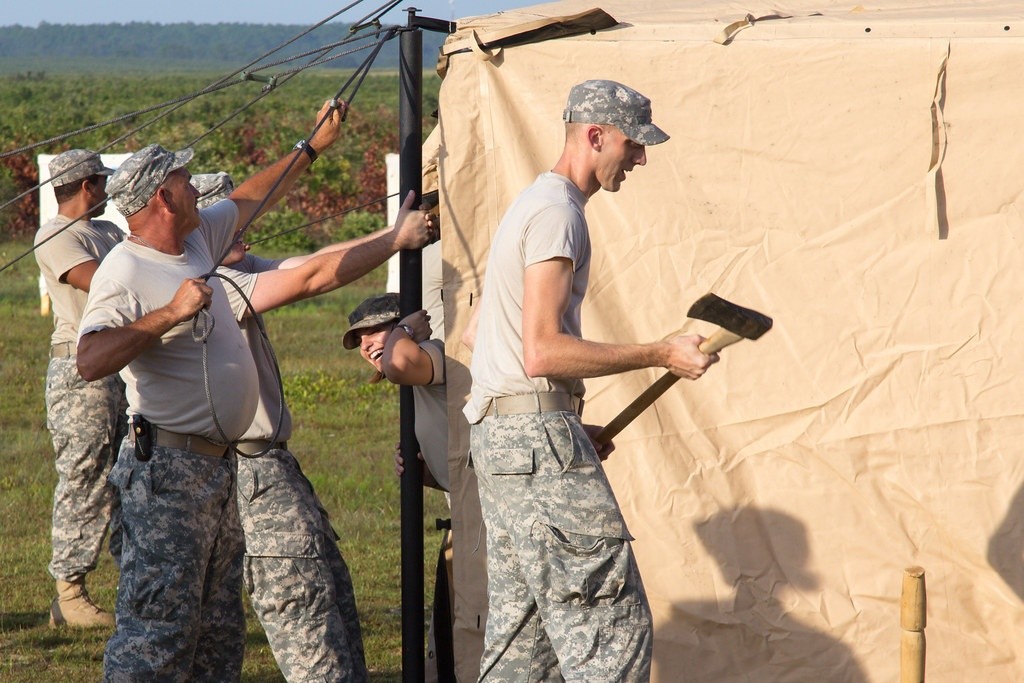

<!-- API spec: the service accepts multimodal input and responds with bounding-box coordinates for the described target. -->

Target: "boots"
[49,579,115,625]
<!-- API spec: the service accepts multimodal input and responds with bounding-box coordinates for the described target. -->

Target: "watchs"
[293,139,318,164]
[399,324,415,338]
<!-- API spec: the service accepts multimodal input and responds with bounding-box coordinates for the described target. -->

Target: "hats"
[563,79,670,146]
[48,149,116,187]
[104,144,194,217]
[342,292,400,350]
[189,171,234,210]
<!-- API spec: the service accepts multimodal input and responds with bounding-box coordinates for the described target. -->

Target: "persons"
[186,172,439,683]
[343,293,450,492]
[32,150,129,627]
[76,99,346,683]
[460,80,720,683]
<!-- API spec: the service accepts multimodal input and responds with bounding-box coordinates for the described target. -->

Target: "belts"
[129,423,287,459]
[485,392,585,416]
[51,342,77,356]
